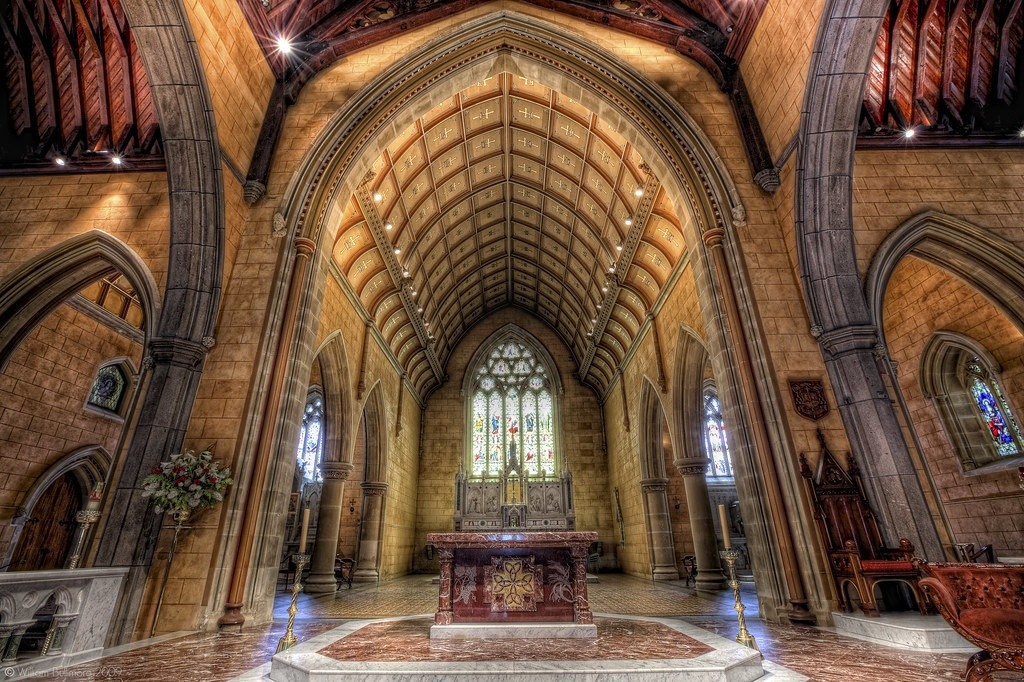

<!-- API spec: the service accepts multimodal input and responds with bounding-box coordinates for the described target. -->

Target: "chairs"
[800,427,937,618]
[681,555,698,586]
[278,553,356,592]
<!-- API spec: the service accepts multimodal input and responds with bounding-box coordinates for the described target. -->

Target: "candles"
[718,504,731,549]
[298,508,311,553]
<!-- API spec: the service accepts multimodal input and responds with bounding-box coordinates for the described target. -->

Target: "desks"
[917,562,1024,682]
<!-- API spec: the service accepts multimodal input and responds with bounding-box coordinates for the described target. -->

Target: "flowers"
[142,448,234,516]
